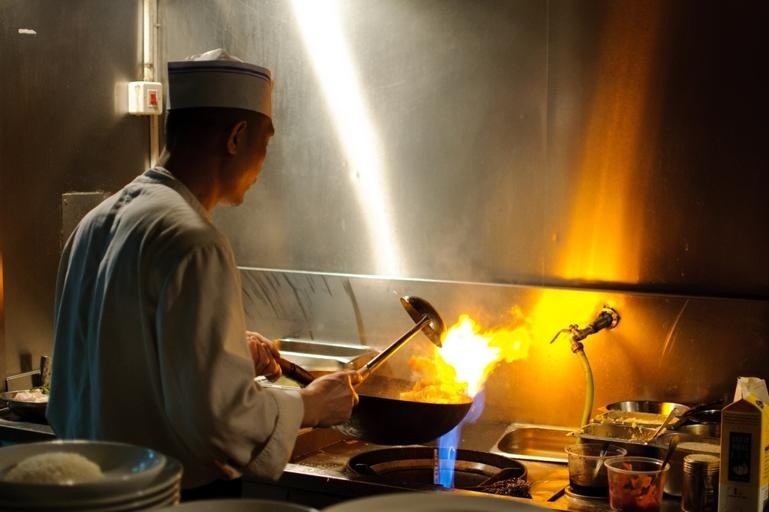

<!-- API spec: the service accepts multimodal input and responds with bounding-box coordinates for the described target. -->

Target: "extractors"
[146,0,769,298]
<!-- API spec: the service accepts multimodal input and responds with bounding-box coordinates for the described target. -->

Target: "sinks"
[487,422,580,465]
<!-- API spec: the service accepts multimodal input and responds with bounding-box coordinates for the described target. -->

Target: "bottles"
[681,454,720,512]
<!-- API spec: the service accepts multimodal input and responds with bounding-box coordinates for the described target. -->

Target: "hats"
[164,47,274,120]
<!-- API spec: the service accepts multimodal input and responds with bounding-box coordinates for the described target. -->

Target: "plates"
[0,440,182,512]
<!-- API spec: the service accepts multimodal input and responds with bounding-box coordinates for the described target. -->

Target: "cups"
[563,443,670,512]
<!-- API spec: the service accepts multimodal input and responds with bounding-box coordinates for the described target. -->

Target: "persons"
[42,45,365,501]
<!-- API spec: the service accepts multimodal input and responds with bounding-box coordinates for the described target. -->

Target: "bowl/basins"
[605,399,691,424]
[1,389,50,417]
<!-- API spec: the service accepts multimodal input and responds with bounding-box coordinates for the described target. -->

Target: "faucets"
[550,307,620,354]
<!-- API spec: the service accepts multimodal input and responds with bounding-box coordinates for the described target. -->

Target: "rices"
[3,451,104,486]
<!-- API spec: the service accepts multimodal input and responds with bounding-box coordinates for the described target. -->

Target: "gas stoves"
[284,445,570,501]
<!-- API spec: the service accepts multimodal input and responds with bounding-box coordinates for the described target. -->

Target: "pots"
[266,343,474,447]
